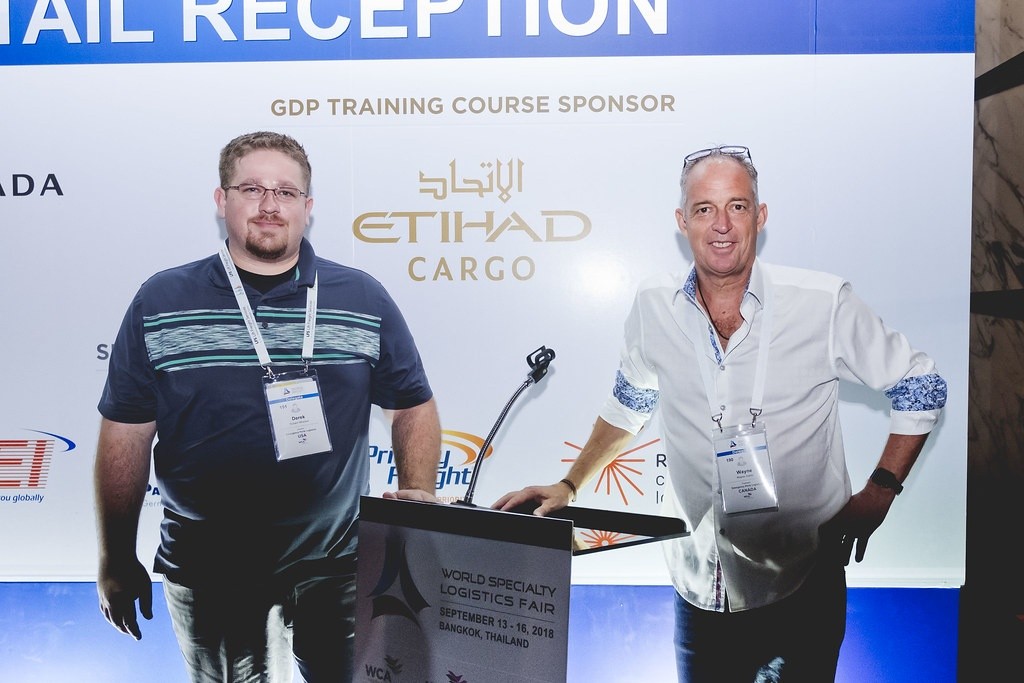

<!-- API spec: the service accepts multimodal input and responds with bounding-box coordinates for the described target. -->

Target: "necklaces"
[697,277,729,340]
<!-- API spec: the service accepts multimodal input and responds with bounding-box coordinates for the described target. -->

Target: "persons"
[95,132,443,683]
[492,145,949,683]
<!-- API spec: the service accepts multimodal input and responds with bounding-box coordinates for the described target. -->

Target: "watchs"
[871,466,904,495]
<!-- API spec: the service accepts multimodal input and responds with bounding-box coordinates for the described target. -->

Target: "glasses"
[683,146,752,170]
[223,183,308,203]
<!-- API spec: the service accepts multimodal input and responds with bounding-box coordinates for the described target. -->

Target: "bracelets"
[559,478,577,502]
